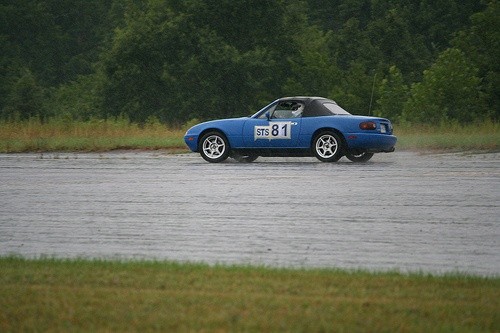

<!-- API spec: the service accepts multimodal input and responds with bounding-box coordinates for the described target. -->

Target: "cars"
[184,96,397,162]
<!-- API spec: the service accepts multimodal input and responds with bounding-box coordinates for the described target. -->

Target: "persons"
[289,101,304,118]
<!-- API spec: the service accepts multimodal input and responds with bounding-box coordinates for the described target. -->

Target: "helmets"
[291,102,305,118]
